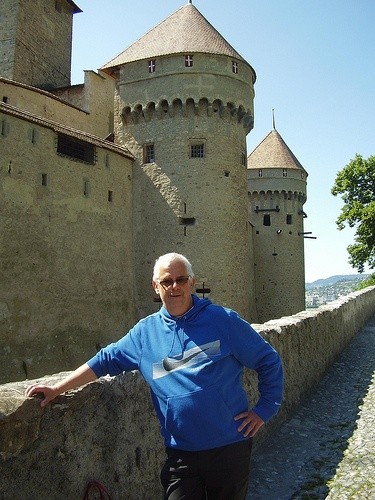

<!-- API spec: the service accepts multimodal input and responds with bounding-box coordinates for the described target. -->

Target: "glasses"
[156,278,189,286]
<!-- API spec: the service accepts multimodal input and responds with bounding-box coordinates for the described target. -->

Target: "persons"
[25,250,284,500]
